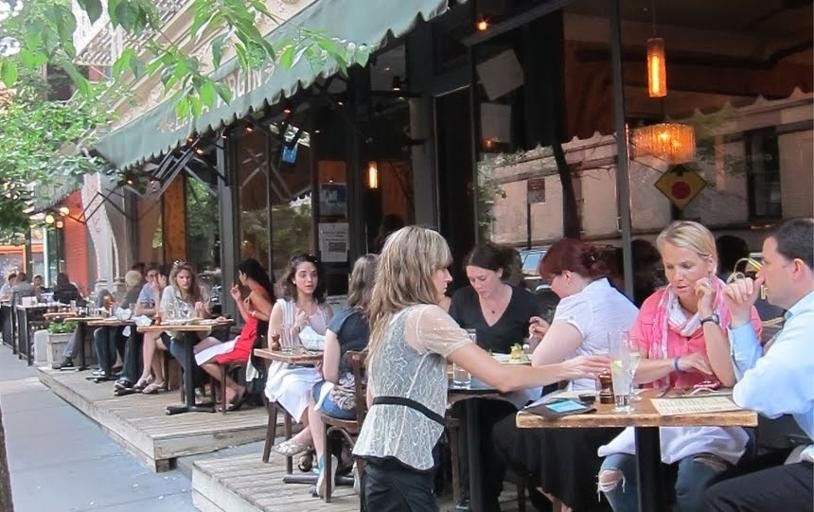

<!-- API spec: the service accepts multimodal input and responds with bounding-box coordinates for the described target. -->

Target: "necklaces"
[481,287,507,314]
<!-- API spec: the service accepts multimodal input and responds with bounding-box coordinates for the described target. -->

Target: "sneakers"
[316,455,339,496]
[51,358,167,394]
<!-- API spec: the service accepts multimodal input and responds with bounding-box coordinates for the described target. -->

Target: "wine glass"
[630,353,643,401]
[103,296,112,318]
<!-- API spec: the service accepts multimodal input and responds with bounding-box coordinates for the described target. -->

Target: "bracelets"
[674,356,680,372]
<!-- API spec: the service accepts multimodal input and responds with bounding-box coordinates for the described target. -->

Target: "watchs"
[701,315,719,324]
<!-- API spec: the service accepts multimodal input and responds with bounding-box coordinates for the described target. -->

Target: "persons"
[51,259,226,394]
[194,257,278,412]
[705,217,814,512]
[2,272,82,332]
[449,242,548,509]
[264,254,380,498]
[351,226,611,512]
[485,237,642,512]
[596,221,763,512]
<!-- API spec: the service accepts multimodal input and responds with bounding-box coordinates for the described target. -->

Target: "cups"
[280,326,301,356]
[466,328,477,345]
[453,361,472,384]
[70,300,76,313]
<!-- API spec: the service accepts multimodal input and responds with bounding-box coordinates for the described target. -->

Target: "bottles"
[607,330,630,409]
[272,329,280,351]
[598,369,615,403]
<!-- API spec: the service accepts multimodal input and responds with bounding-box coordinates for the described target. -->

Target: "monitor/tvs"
[277,142,300,167]
[520,250,546,280]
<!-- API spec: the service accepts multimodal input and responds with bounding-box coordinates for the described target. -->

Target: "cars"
[517,245,549,286]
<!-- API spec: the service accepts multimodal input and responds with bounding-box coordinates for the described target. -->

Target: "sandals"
[297,452,314,472]
[271,439,313,457]
[335,461,355,476]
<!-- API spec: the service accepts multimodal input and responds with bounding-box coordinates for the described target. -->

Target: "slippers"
[219,392,249,411]
